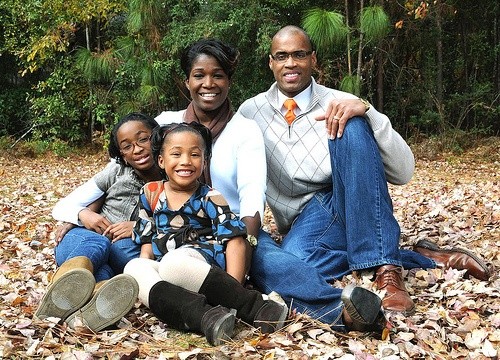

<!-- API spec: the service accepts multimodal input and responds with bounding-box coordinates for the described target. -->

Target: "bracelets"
[364,99,369,112]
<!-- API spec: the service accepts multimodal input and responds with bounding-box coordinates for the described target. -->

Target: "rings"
[334,116,340,120]
[244,274,250,280]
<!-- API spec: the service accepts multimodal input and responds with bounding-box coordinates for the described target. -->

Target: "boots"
[31,255,95,326]
[64,273,140,335]
[147,280,236,346]
[198,265,289,335]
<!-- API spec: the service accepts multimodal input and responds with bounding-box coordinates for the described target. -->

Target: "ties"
[282,99,298,124]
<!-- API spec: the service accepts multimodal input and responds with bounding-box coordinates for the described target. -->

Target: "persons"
[237,25,490,315]
[35,111,166,335]
[54,39,393,340]
[123,121,288,347]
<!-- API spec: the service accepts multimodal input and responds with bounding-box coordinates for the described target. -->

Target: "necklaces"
[192,102,227,131]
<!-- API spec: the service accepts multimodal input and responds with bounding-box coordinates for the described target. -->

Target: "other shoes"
[340,284,385,335]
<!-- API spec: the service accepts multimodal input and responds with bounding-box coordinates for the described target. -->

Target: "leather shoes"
[373,265,415,316]
[414,240,490,281]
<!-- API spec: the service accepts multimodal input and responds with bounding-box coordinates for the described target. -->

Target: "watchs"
[246,234,258,247]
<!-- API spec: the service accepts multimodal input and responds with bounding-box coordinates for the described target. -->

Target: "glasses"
[270,50,313,61]
[120,135,151,154]
